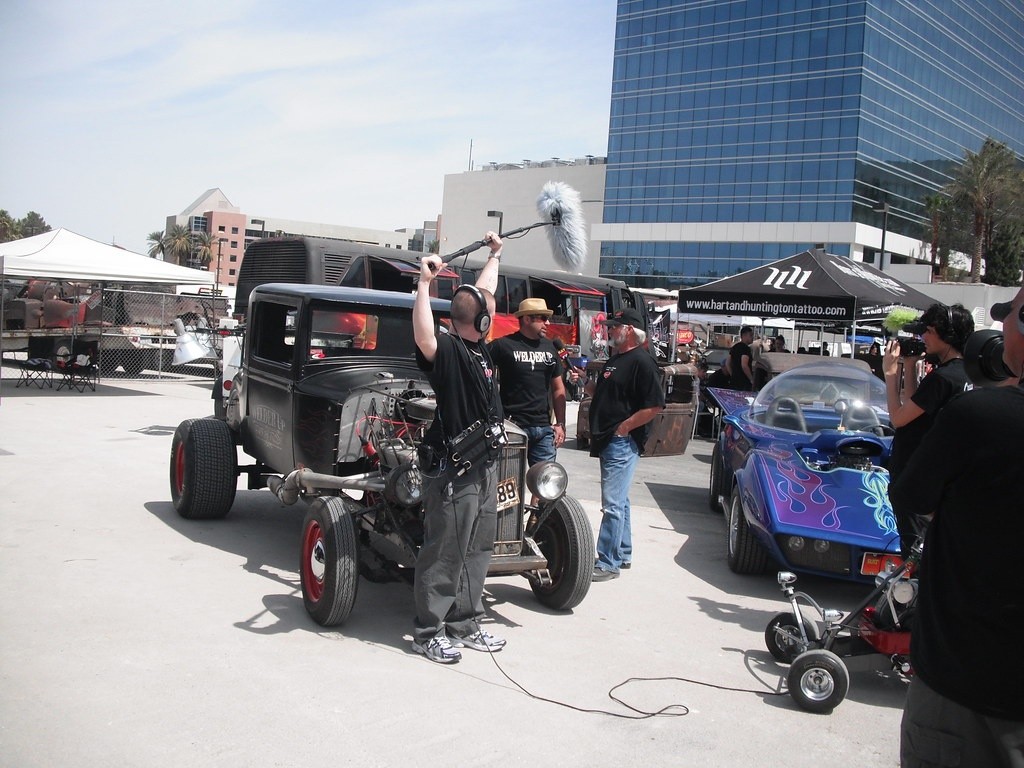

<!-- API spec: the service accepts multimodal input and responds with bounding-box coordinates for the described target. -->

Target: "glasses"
[613,324,628,330]
[529,315,549,322]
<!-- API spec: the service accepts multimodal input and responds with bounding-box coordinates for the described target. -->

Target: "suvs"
[169,283,595,627]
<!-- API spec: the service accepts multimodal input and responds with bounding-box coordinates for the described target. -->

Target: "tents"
[671,247,944,359]
[0,228,219,380]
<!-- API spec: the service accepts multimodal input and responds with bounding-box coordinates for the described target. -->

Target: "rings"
[559,437,563,440]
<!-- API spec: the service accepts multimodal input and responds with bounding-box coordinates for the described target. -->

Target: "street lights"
[251,219,265,239]
[873,202,889,271]
[217,238,229,292]
[488,211,503,240]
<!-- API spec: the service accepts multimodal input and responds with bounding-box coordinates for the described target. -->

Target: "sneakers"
[412,634,462,664]
[594,558,631,569]
[591,566,620,582]
[445,627,506,651]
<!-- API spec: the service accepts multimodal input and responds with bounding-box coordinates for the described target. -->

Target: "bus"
[232,235,650,388]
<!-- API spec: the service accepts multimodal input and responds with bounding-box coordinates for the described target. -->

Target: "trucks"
[27,276,178,375]
[144,286,229,370]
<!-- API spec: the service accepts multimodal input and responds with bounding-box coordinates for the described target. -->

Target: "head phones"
[945,305,959,345]
[453,283,491,333]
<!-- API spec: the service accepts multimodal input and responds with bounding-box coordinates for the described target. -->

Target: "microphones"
[552,338,587,388]
[535,181,589,272]
[902,323,928,336]
[990,303,1012,322]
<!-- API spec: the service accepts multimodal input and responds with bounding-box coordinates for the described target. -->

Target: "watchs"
[489,251,501,259]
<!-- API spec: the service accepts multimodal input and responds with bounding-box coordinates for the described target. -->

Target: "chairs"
[765,396,808,433]
[16,335,99,395]
[843,406,884,437]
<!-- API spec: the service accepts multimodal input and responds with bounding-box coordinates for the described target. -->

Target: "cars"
[706,361,915,588]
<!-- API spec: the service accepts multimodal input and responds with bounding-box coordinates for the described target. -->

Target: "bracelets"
[557,421,566,429]
[584,376,589,385]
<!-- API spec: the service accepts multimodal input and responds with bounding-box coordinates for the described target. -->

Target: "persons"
[568,306,666,582]
[413,230,508,664]
[697,325,830,441]
[865,287,1024,768]
[486,298,567,531]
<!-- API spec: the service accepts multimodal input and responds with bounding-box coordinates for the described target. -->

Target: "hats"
[513,298,553,319]
[600,308,644,331]
